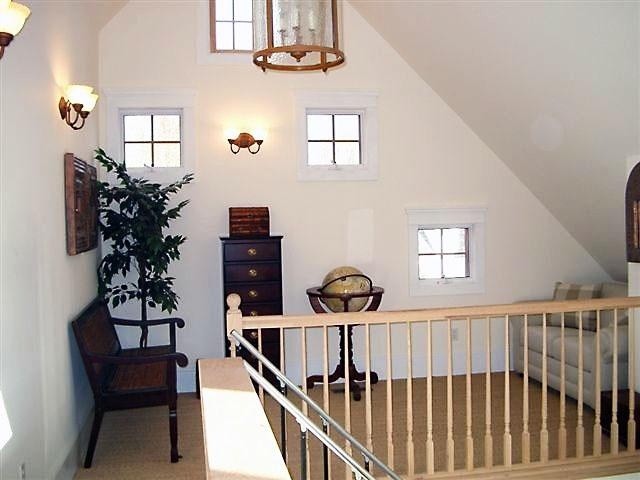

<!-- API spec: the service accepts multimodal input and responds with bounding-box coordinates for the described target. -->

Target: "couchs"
[508,280,628,411]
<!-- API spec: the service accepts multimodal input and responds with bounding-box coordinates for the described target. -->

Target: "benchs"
[70,295,190,470]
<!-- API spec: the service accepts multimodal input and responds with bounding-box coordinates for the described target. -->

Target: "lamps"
[57,82,99,133]
[250,0,347,76]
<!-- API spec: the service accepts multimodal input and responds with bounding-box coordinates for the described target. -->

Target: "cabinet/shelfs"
[218,235,287,395]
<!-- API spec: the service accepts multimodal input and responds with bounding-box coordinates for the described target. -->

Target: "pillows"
[545,281,604,332]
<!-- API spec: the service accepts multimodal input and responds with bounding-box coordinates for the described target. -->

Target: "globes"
[306,266,385,402]
